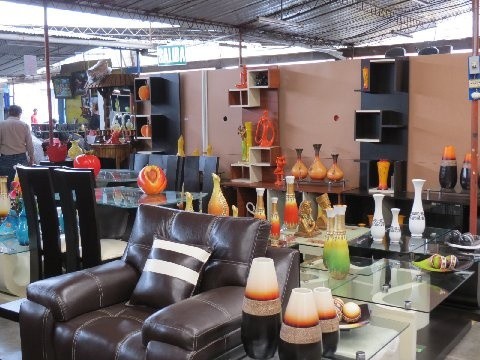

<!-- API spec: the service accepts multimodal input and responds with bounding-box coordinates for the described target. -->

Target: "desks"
[221,180,353,217]
[341,188,470,233]
[91,138,131,169]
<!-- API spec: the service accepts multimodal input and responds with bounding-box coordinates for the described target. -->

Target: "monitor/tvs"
[52,76,74,99]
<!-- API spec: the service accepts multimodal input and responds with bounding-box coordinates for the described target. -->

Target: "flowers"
[9,180,25,203]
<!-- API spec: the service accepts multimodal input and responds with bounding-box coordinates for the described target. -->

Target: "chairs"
[16,161,82,284]
[50,165,129,279]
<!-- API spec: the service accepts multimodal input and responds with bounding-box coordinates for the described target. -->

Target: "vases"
[253,188,265,221]
[326,153,345,183]
[389,207,402,243]
[208,173,229,219]
[270,197,280,239]
[291,149,308,181]
[19,197,31,247]
[68,140,83,160]
[439,146,457,193]
[409,178,426,236]
[315,288,340,357]
[328,205,350,280]
[376,160,390,190]
[460,153,472,193]
[370,194,386,240]
[322,207,334,269]
[278,287,323,360]
[284,176,300,229]
[307,143,328,182]
[362,66,369,88]
[244,121,252,163]
[241,258,280,360]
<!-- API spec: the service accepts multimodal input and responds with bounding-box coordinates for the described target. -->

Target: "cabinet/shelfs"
[297,251,474,359]
[353,56,407,197]
[348,225,480,323]
[230,145,281,185]
[131,77,167,151]
[226,67,280,110]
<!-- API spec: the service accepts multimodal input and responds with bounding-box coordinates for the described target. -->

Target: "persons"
[30,108,40,125]
[0,105,35,192]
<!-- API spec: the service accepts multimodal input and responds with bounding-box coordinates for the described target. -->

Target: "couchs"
[18,203,300,360]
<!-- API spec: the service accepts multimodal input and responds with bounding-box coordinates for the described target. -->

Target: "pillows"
[124,233,215,311]
